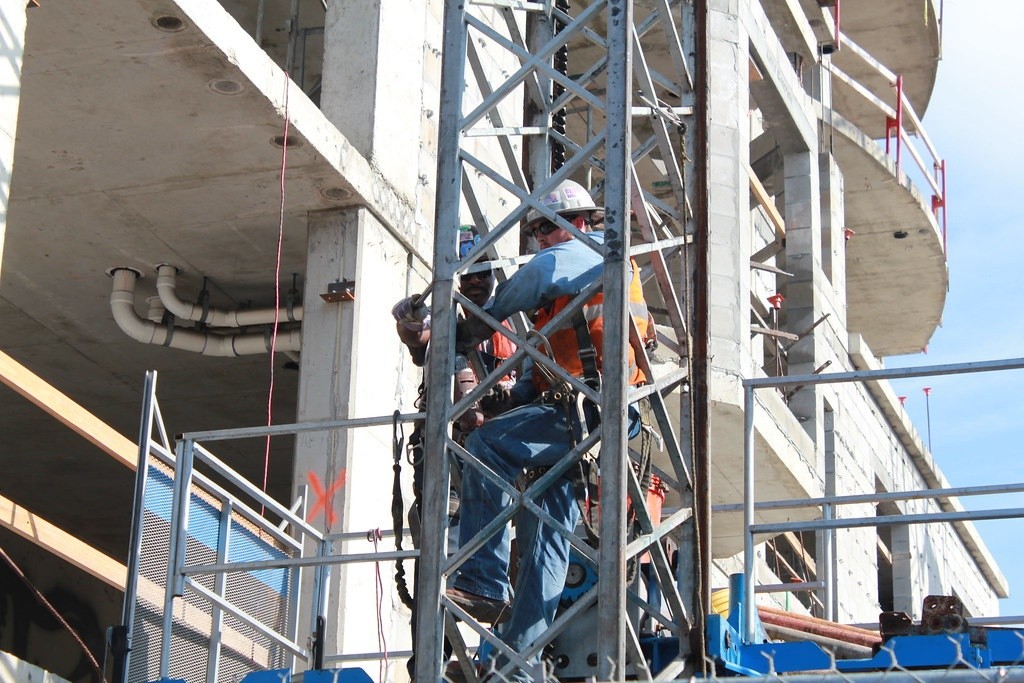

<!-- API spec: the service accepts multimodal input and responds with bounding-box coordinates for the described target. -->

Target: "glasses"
[526,214,578,239]
[461,269,492,281]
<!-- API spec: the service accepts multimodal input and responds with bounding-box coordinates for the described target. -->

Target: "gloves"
[392,293,429,330]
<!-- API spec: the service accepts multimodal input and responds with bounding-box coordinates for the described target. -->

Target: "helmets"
[459,224,489,262]
[518,180,605,237]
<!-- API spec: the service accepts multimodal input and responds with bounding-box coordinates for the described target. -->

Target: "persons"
[394,223,524,595]
[441,179,653,683]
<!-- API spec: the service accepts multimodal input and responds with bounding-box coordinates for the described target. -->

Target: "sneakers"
[445,588,512,624]
[445,659,493,683]
